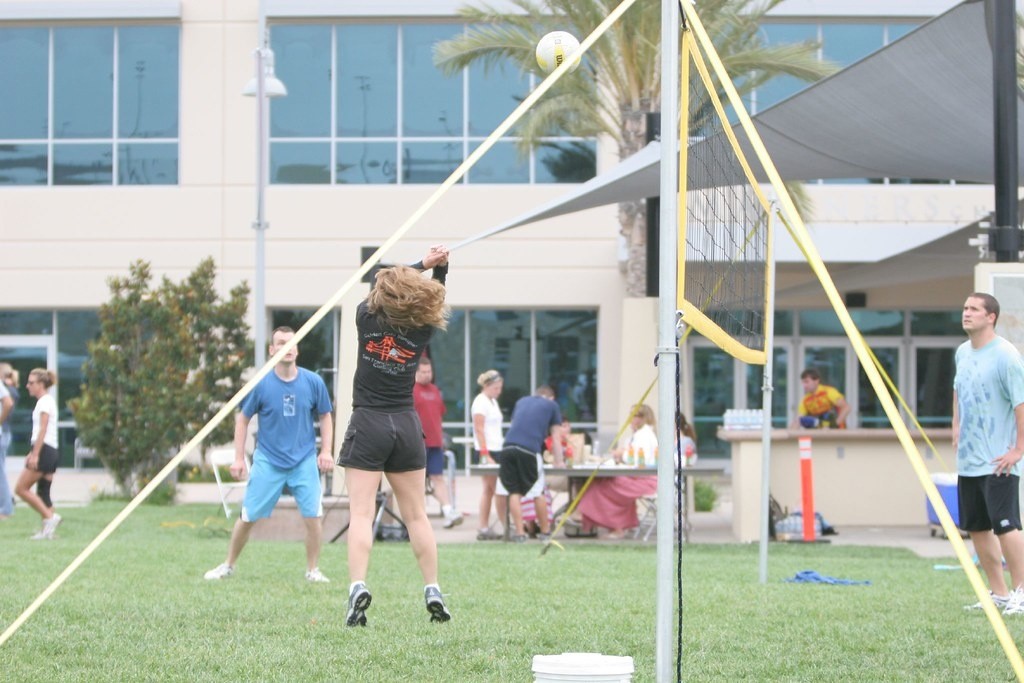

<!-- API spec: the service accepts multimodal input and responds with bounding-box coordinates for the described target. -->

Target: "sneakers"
[1002,588,1024,615]
[964,591,1014,610]
[424,583,451,623]
[343,580,372,626]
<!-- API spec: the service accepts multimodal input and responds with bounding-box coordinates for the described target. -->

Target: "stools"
[72,438,102,479]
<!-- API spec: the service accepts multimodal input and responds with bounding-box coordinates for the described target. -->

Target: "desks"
[469,463,725,540]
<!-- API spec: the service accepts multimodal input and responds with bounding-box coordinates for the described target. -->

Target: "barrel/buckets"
[530,653,635,682]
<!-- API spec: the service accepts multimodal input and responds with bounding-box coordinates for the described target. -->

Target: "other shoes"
[305,567,329,584]
[29,532,51,540]
[515,533,529,543]
[42,513,61,535]
[204,563,236,581]
[443,509,464,529]
[600,531,624,540]
[539,531,551,543]
[476,528,502,541]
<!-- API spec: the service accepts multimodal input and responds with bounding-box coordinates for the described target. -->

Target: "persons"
[950,291,1024,620]
[460,367,564,544]
[16,366,65,541]
[558,400,659,538]
[201,325,332,586]
[795,369,851,430]
[408,358,466,530]
[335,243,453,628]
[674,411,698,468]
[0,358,19,518]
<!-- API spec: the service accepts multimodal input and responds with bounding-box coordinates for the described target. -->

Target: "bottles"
[724,408,763,431]
[627,443,658,470]
[773,512,822,537]
[561,441,573,469]
[684,442,694,467]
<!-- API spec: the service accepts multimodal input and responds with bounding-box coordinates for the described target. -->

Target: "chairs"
[635,495,659,540]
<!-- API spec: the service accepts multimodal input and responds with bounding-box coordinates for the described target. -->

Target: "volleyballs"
[535,31,582,74]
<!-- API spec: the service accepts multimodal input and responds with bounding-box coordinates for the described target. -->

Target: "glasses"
[27,381,34,385]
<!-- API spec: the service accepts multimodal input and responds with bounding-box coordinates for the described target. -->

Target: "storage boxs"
[926,471,970,539]
